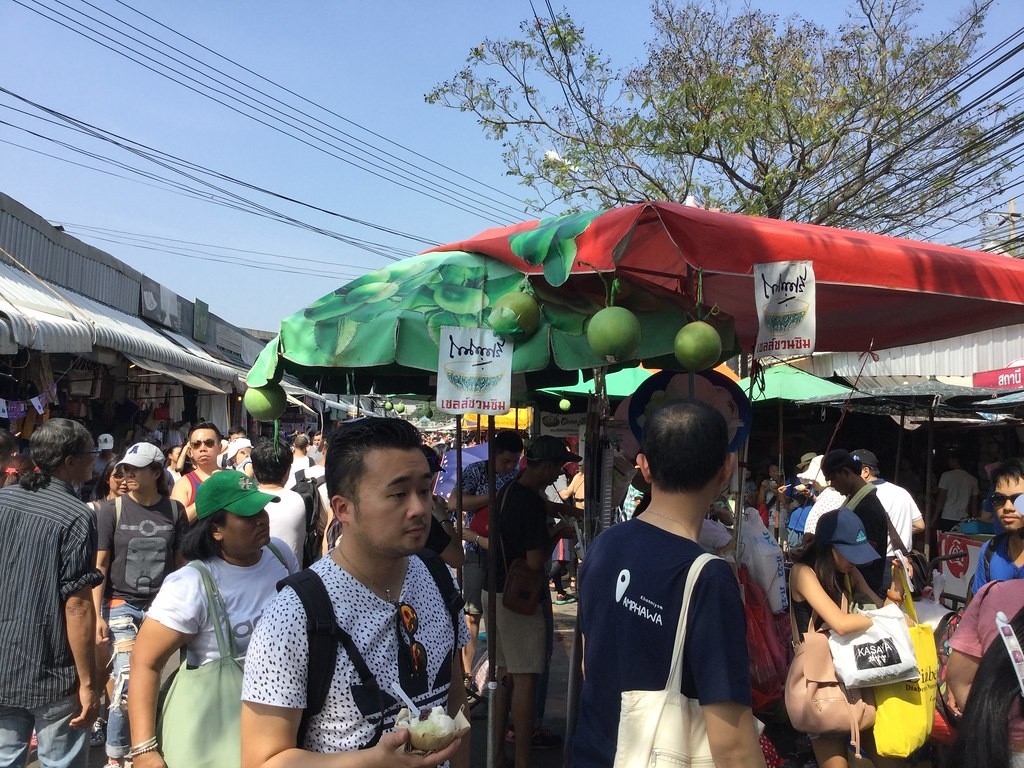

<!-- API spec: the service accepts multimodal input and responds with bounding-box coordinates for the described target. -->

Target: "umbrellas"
[539,368,654,399]
[243,200,1024,767]
[804,375,1024,573]
[736,364,855,547]
[433,441,488,503]
[971,391,1024,416]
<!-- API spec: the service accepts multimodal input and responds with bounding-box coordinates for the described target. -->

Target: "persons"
[0,401,1024,768]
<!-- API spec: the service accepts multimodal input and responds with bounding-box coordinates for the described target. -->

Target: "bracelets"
[440,519,450,523]
[131,743,158,756]
[130,736,156,752]
[134,740,156,752]
[887,590,902,601]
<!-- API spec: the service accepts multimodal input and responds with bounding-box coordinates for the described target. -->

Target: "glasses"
[111,472,125,479]
[71,446,103,458]
[190,439,217,450]
[393,601,428,683]
[990,491,1024,507]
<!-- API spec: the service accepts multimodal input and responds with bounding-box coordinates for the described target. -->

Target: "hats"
[114,442,165,469]
[815,507,881,565]
[97,433,114,449]
[821,448,853,473]
[226,437,255,460]
[195,471,280,522]
[796,452,816,469]
[849,449,879,466]
[419,444,446,473]
[525,434,583,463]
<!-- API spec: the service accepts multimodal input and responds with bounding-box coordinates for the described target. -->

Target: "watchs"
[472,536,479,547]
[809,495,814,500]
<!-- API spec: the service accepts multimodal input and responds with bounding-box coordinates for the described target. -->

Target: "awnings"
[0,260,371,416]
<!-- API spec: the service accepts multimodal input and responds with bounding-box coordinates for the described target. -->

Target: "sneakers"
[506,724,563,749]
[88,716,108,746]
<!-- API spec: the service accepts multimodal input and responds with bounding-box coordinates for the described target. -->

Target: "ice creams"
[399,705,454,737]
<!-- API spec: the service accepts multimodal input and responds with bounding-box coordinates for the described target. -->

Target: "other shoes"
[103,756,126,768]
[29,729,38,754]
[557,593,575,601]
[463,673,475,699]
[477,632,488,641]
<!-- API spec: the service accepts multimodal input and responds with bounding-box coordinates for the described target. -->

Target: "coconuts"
[559,398,570,411]
[587,306,641,363]
[244,379,287,422]
[674,320,722,372]
[385,402,434,418]
[490,291,540,337]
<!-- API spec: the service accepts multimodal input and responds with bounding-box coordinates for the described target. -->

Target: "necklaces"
[647,511,693,536]
[339,545,405,595]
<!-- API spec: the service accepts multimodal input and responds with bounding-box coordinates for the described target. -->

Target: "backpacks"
[289,468,325,570]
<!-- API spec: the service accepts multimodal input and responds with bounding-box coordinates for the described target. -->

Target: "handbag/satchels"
[784,548,941,755]
[738,507,795,711]
[156,558,248,768]
[503,560,544,615]
[612,553,765,768]
